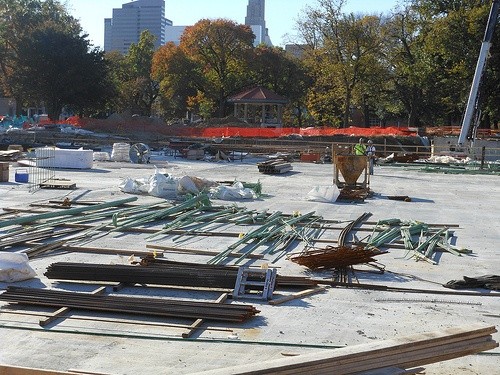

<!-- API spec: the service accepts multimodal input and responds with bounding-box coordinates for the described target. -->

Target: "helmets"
[367,140,374,144]
[359,138,365,143]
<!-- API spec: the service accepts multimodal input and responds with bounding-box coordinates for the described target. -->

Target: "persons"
[366,140,376,175]
[354,138,366,155]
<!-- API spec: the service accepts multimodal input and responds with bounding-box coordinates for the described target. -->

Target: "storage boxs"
[15,173,28,183]
